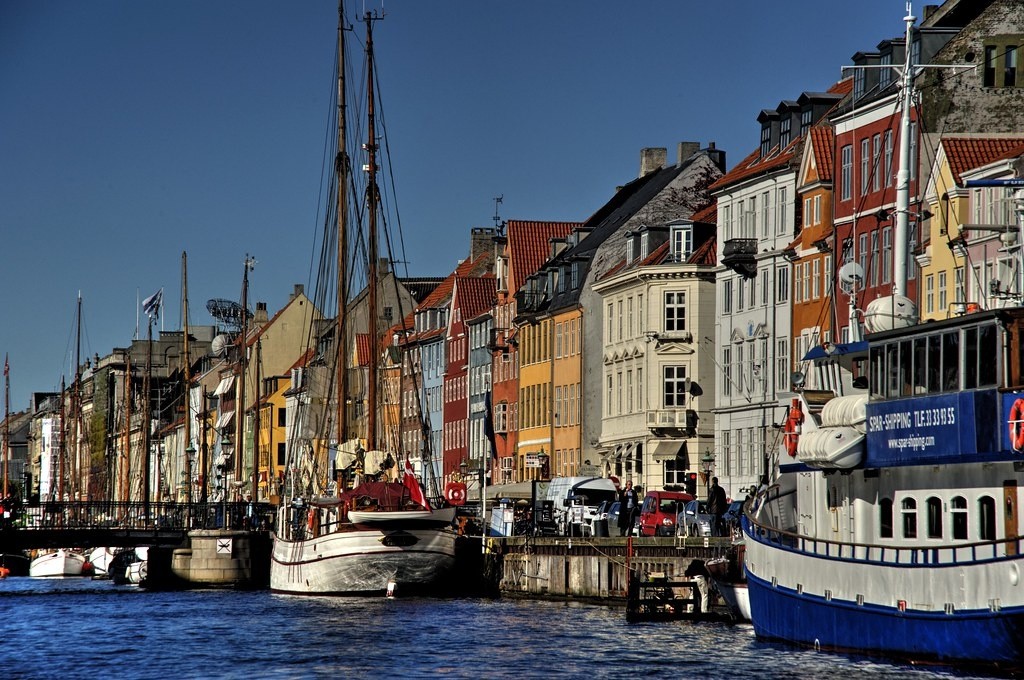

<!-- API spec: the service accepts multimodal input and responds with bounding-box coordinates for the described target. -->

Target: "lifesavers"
[309,509,314,530]
[338,500,350,510]
[1009,397,1024,450]
[784,416,798,457]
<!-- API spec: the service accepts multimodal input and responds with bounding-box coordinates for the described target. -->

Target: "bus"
[546,477,618,536]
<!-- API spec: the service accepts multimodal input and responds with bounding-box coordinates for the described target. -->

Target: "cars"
[677,500,745,537]
[590,501,643,536]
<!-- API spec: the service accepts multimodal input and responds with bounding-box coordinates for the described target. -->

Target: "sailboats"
[0,0,487,598]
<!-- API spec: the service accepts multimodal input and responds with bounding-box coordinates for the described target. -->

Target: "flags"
[403,462,434,514]
[142,290,163,313]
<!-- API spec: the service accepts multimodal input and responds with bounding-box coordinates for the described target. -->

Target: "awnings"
[652,439,685,463]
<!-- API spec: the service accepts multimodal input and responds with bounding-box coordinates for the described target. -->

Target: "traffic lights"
[479,468,484,483]
[683,472,697,495]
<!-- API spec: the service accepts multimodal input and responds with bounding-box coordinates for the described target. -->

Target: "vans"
[639,491,694,537]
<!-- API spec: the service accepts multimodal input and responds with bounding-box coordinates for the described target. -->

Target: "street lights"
[537,446,549,481]
[702,447,715,499]
[459,455,468,484]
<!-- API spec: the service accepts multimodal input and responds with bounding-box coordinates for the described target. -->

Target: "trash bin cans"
[593,520,609,537]
[568,522,582,537]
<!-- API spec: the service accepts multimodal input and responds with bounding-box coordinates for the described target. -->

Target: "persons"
[159,486,270,532]
[620,475,771,538]
[0,491,19,529]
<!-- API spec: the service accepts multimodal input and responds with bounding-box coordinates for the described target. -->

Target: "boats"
[710,0,1024,673]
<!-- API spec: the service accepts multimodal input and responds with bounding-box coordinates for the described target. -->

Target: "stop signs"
[607,476,620,490]
